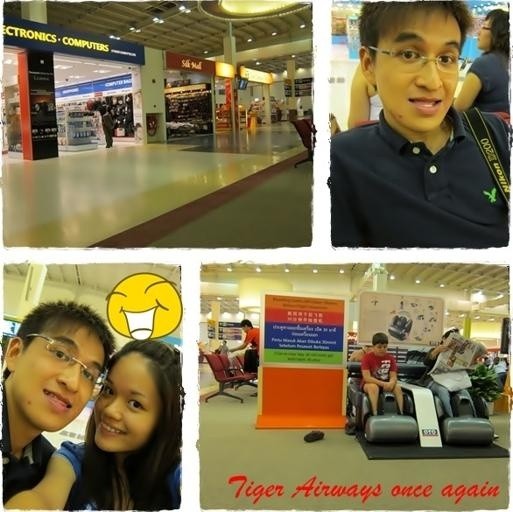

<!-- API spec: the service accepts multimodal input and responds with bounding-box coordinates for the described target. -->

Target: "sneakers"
[305,431,325,441]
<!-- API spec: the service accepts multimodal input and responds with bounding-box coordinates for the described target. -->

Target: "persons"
[330,0,509,249]
[419,328,478,418]
[2,338,184,511]
[347,61,384,130]
[452,9,510,124]
[228,320,259,359]
[359,332,404,416]
[102,107,116,148]
[3,300,116,506]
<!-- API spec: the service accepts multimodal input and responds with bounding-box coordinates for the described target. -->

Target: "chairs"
[202,351,258,404]
[289,119,316,167]
[348,362,494,445]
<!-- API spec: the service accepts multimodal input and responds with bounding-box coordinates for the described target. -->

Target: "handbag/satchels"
[103,112,114,129]
[244,349,257,373]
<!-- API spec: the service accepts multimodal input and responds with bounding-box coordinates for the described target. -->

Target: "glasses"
[368,45,465,72]
[26,333,103,384]
[481,24,492,30]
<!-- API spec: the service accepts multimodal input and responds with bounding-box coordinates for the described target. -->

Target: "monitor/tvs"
[239,79,248,90]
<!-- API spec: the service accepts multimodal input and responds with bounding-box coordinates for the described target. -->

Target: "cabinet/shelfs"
[3,83,212,156]
